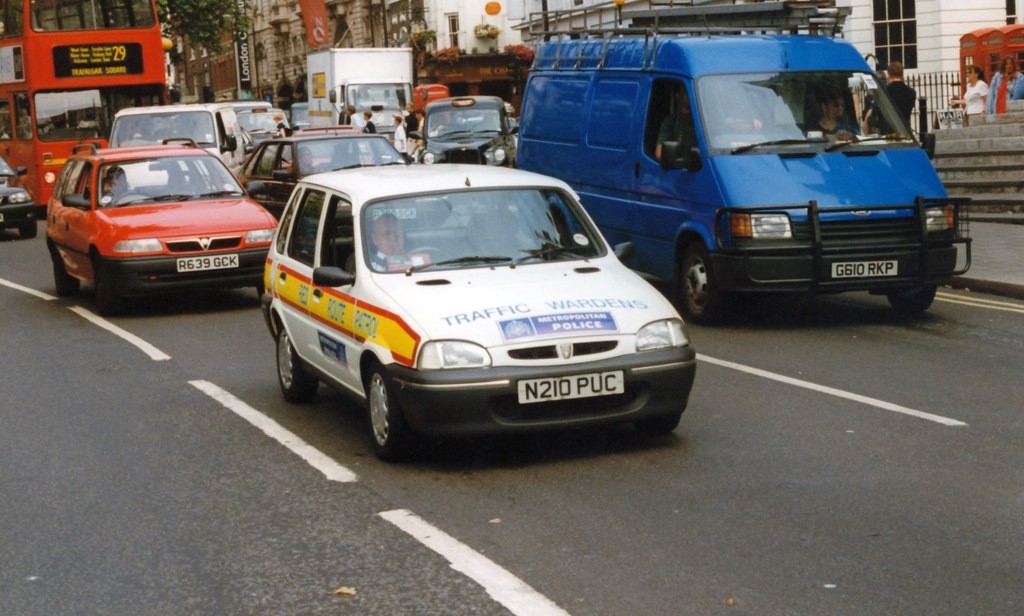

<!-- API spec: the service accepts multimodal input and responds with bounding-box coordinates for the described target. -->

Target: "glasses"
[833,99,848,109]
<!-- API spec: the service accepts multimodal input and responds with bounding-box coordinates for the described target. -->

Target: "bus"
[0,0,169,206]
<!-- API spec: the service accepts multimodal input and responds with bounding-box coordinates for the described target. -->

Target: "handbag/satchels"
[280,123,293,137]
[937,105,963,129]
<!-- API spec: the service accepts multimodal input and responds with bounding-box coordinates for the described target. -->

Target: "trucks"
[307,48,414,145]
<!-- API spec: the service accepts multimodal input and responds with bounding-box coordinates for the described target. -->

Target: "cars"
[261,162,696,464]
[407,96,520,170]
[0,156,37,238]
[45,137,278,317]
[232,101,307,141]
[237,132,452,228]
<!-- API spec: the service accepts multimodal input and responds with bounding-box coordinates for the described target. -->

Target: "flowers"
[475,24,500,38]
[504,44,534,64]
[434,47,459,66]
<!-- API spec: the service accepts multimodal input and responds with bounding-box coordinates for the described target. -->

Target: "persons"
[392,101,425,161]
[361,111,375,133]
[863,60,916,134]
[272,114,287,137]
[805,86,845,133]
[339,105,356,125]
[286,147,325,177]
[368,213,435,272]
[947,56,1024,116]
[106,166,136,203]
[655,82,705,161]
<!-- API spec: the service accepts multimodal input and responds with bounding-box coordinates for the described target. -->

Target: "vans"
[107,101,246,197]
[516,30,972,327]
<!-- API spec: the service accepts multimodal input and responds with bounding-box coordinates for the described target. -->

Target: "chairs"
[457,208,522,260]
